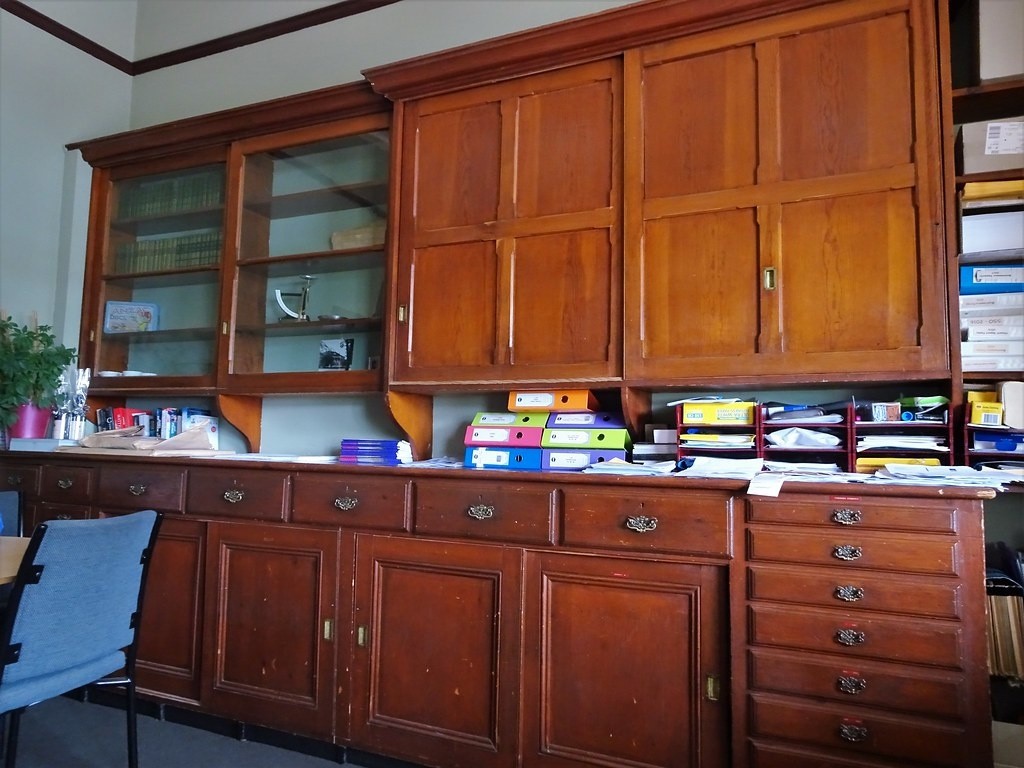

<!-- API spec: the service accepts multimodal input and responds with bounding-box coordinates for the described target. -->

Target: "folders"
[541,449,629,471]
[471,411,550,428]
[959,263,1024,372]
[507,388,600,413]
[463,425,543,448]
[540,428,633,453]
[463,447,543,471]
[546,412,627,429]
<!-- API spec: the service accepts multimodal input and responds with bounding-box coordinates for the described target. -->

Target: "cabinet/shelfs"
[0,1,1024,768]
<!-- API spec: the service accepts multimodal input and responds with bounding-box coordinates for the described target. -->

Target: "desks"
[0,536,32,585]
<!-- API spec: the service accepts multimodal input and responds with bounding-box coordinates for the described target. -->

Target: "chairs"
[0,509,165,767]
[0,489,24,615]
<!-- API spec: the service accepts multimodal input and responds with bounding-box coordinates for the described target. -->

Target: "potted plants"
[0,315,82,440]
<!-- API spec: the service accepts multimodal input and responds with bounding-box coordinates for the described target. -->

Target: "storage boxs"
[954,117,1024,175]
[977,0,1024,86]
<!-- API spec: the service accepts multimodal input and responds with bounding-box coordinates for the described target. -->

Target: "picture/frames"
[104,300,158,332]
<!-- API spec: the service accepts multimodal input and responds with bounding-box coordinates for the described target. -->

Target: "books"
[631,426,755,464]
[114,164,226,273]
[93,404,217,447]
[855,434,951,453]
[339,438,413,466]
